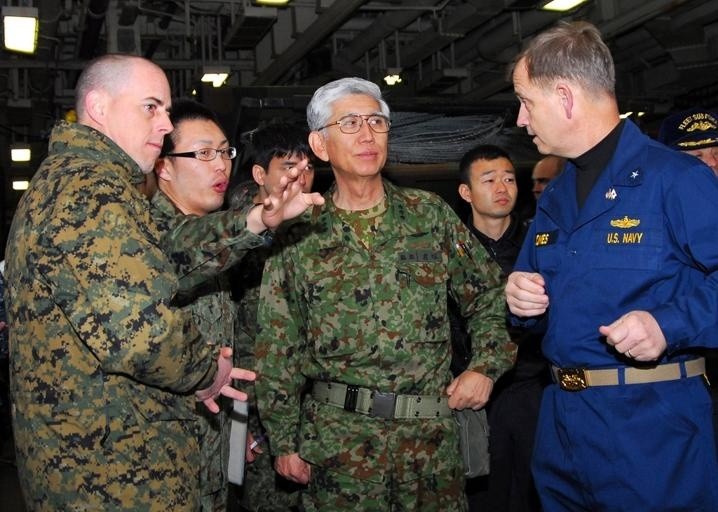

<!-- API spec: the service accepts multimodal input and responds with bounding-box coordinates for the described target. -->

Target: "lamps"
[0,2,41,192]
[193,15,233,88]
[378,28,404,87]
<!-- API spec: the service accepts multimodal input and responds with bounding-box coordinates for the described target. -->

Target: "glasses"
[159,144,237,164]
[313,112,392,137]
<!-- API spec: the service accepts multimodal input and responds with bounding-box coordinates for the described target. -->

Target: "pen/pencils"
[455,243,474,261]
[252,435,266,450]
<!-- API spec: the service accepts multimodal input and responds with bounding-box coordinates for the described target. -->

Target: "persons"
[450,145,541,512]
[0,54,259,512]
[254,75,523,512]
[221,121,327,512]
[652,107,718,195]
[527,156,572,208]
[154,93,327,512]
[500,18,716,511]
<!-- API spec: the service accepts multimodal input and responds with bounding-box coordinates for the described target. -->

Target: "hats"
[660,109,718,152]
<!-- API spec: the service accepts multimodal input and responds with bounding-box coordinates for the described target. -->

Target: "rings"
[628,350,636,358]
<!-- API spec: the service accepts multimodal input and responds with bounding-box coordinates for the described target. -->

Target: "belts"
[547,356,708,392]
[308,374,460,424]
